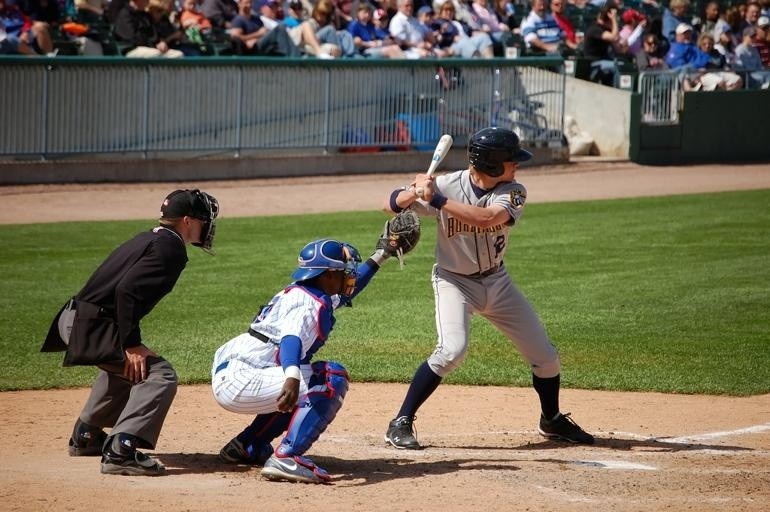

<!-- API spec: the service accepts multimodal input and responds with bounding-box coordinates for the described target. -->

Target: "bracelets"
[370,253,385,267]
[429,193,447,211]
[285,365,301,380]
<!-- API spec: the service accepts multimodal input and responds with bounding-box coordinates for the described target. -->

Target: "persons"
[41,188,220,476]
[384,127,595,449]
[211,211,421,484]
[0,1,769,93]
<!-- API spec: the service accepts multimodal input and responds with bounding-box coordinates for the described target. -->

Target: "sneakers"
[99,441,165,476]
[538,411,595,445]
[219,433,274,465]
[384,416,421,450]
[68,430,110,457]
[261,452,325,484]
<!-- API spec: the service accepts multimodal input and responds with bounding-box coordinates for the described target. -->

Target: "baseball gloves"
[377,210,420,256]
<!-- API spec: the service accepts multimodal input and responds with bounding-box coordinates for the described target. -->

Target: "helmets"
[469,127,533,177]
[291,239,363,302]
[159,188,219,250]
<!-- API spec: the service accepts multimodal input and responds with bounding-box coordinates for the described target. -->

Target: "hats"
[623,10,646,22]
[419,7,435,17]
[676,23,693,34]
[260,1,279,9]
[289,0,303,10]
[373,9,388,20]
[743,27,757,38]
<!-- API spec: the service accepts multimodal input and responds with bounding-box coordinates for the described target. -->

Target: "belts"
[67,300,77,310]
[247,327,274,346]
[469,260,503,276]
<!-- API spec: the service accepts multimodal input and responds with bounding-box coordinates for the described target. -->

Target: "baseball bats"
[416,134,453,197]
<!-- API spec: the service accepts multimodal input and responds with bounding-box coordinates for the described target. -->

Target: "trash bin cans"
[396,94,440,151]
